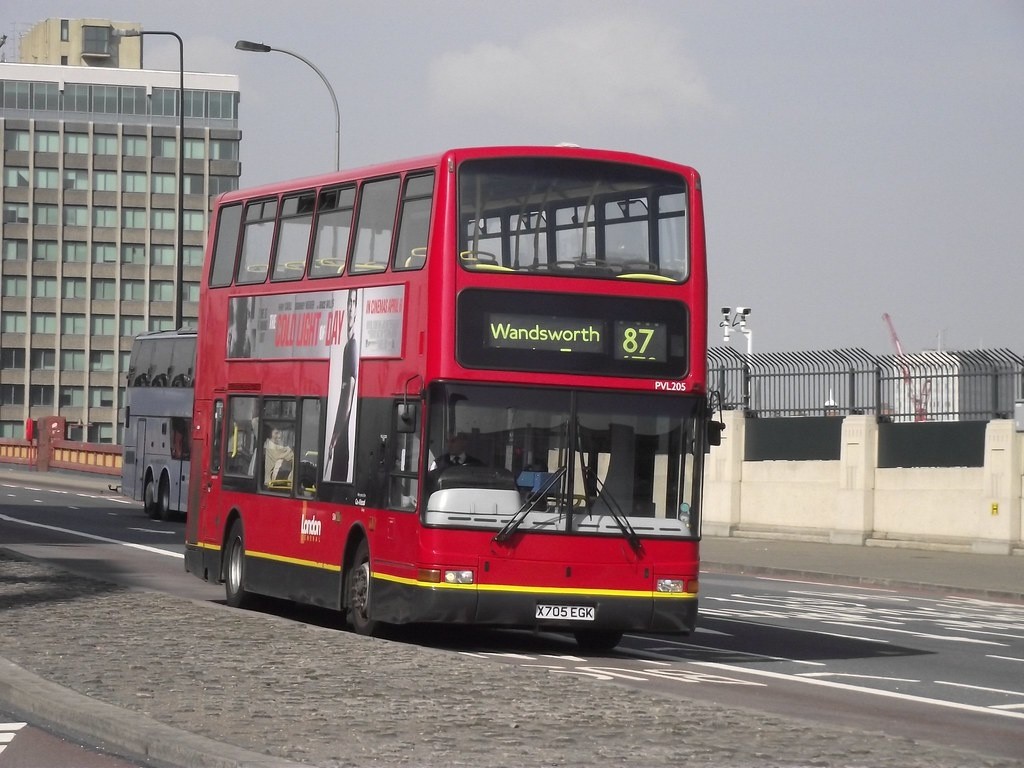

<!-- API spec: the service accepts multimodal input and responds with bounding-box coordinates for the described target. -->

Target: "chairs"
[216,245,686,286]
[216,415,560,514]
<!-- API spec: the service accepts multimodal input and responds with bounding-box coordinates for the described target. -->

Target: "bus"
[118,327,198,521]
[184,144,727,649]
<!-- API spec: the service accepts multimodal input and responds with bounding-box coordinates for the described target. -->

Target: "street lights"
[111,29,183,329]
[234,39,340,173]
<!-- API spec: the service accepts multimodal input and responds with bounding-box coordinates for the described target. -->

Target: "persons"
[328,288,358,482]
[429,430,484,472]
[247,424,294,483]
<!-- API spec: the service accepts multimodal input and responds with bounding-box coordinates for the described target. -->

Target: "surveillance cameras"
[721,307,730,315]
[736,307,752,316]
